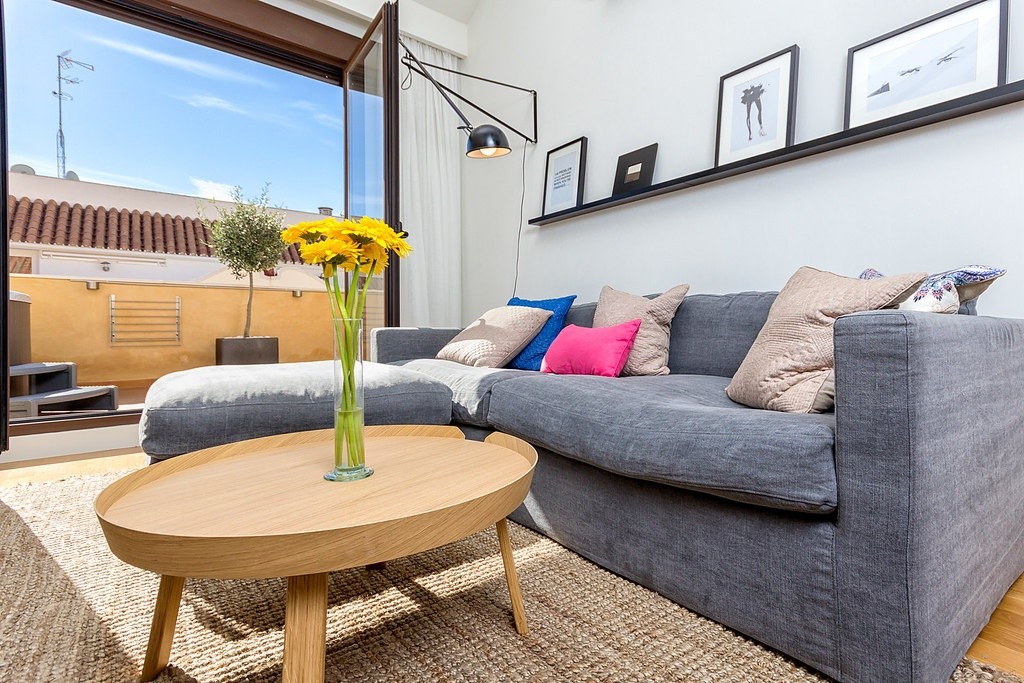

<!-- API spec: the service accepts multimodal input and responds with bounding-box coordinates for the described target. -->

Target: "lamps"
[100,262,111,272]
[397,37,538,159]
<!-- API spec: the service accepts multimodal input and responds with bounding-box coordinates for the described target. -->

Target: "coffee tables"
[94,424,537,683]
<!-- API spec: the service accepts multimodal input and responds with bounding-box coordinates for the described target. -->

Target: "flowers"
[278,215,415,466]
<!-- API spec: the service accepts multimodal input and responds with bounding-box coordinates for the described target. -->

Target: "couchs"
[139,294,1024,683]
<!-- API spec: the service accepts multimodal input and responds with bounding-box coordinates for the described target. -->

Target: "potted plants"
[193,180,290,365]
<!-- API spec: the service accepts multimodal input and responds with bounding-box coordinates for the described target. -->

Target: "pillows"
[592,284,689,376]
[506,295,578,372]
[434,305,554,368]
[540,320,641,378]
[726,266,1006,415]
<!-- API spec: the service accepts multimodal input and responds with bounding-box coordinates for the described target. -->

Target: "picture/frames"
[843,0,1009,131]
[541,136,588,217]
[611,143,659,195]
[714,44,800,168]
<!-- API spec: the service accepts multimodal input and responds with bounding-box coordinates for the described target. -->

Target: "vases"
[322,318,375,483]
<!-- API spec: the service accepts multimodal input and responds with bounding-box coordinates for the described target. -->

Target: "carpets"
[0,464,1024,683]
